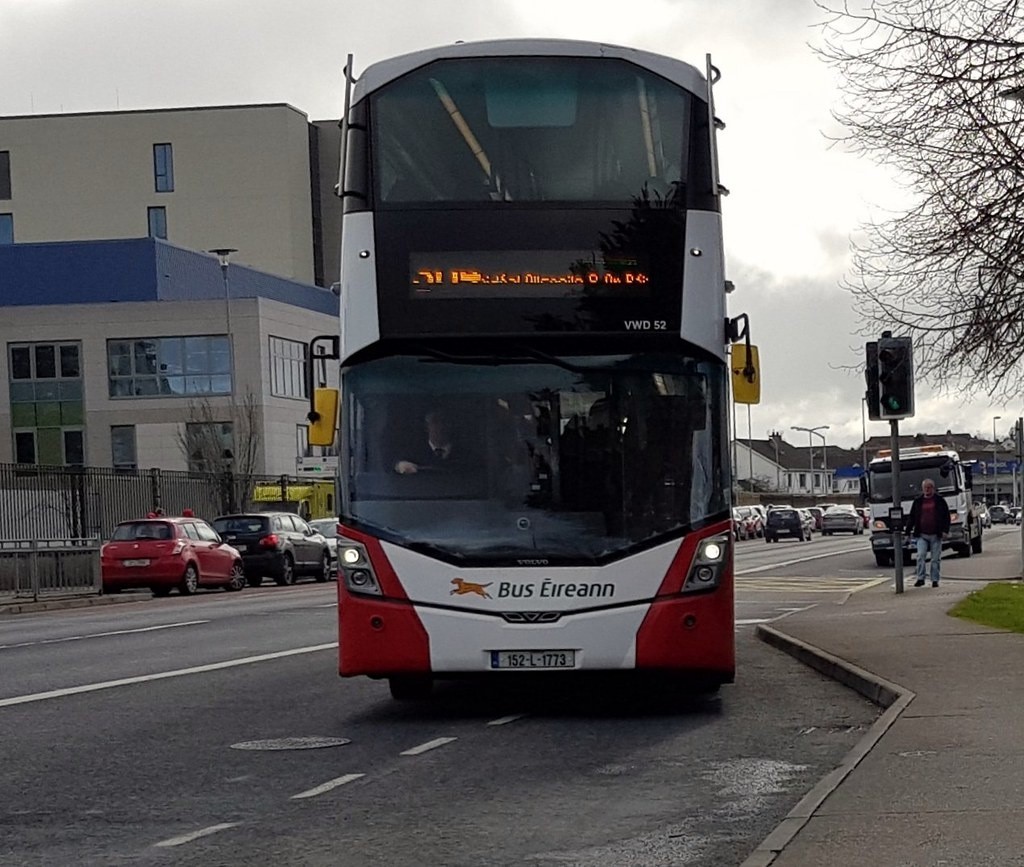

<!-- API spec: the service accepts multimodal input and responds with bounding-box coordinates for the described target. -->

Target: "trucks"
[866,444,987,570]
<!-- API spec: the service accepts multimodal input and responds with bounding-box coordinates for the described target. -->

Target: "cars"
[856,508,871,529]
[801,503,837,533]
[210,511,333,587]
[307,517,339,575]
[729,504,794,541]
[973,501,1022,528]
[819,505,866,535]
[97,509,246,595]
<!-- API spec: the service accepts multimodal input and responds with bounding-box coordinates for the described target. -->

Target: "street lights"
[808,425,829,495]
[979,461,991,508]
[993,415,1000,505]
[791,426,829,494]
[1010,463,1019,508]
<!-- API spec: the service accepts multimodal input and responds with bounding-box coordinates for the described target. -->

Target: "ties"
[436,449,444,458]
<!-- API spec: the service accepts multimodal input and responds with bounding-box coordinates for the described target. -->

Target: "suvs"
[764,508,815,541]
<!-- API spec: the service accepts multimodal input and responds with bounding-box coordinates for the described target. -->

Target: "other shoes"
[914,580,925,586]
[932,581,939,587]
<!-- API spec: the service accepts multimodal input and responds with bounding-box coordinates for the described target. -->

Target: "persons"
[394,405,471,476]
[905,479,951,588]
[145,508,163,519]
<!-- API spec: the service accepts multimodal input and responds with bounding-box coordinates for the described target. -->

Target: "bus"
[301,37,762,713]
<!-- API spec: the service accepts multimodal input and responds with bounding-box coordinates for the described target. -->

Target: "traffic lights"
[878,338,920,430]
